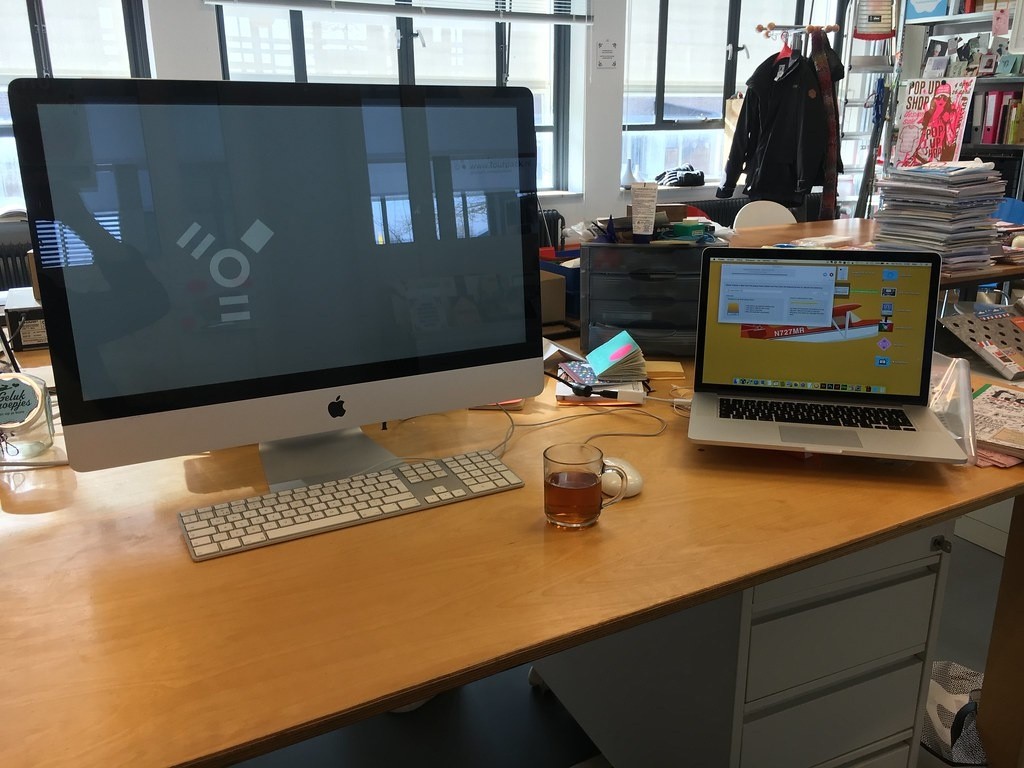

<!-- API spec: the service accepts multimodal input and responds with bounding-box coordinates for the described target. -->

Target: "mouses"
[601,458,643,498]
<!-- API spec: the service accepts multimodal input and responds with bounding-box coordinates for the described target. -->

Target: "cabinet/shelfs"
[581,245,727,355]
[884,0,1024,162]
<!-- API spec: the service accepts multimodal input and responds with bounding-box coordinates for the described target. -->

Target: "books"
[972,90,1024,145]
[556,330,686,403]
[869,157,1024,272]
[470,398,525,411]
[972,384,1024,459]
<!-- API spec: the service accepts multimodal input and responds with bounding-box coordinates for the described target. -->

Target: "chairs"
[941,197,1024,317]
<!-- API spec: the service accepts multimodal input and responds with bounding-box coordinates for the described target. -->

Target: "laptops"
[687,248,968,464]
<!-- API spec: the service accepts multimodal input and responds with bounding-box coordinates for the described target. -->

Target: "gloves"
[655,163,704,186]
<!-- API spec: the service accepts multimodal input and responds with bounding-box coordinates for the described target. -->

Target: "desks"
[0,346,1024,767]
[726,219,1023,290]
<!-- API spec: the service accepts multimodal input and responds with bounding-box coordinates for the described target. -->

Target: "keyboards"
[176,449,525,561]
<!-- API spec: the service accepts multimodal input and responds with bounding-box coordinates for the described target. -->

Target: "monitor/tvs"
[7,77,545,495]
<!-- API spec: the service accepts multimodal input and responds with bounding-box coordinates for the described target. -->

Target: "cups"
[542,443,628,532]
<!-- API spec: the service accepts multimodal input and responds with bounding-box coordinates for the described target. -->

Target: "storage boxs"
[627,204,688,222]
[27,249,42,300]
[540,249,581,323]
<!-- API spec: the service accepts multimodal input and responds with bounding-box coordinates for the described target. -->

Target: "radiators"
[680,193,840,229]
[0,240,32,290]
[539,210,566,249]
